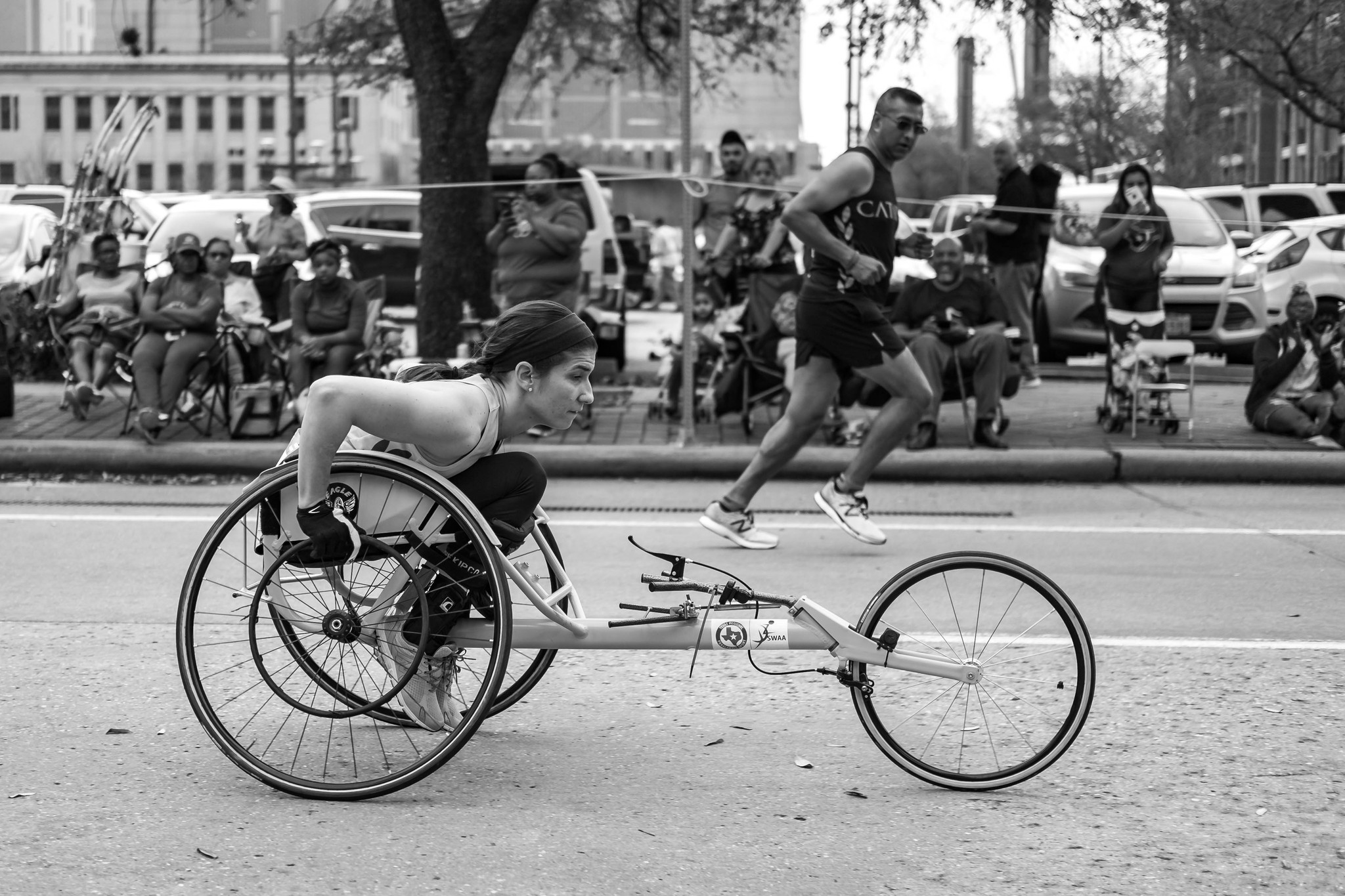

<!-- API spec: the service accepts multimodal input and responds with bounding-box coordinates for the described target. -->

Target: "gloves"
[297,497,368,565]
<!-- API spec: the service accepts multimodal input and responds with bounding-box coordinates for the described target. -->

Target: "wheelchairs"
[172,438,1099,804]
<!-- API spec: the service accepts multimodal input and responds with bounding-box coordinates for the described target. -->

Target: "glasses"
[881,111,928,140]
[210,252,227,257]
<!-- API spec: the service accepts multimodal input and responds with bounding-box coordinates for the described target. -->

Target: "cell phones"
[1126,186,1150,216]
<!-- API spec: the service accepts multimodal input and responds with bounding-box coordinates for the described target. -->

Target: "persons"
[487,150,591,449]
[965,141,1047,389]
[263,299,599,734]
[30,176,404,437]
[1015,136,1065,358]
[660,139,1024,453]
[1093,164,1176,425]
[1244,280,1345,447]
[644,219,685,315]
[695,86,941,552]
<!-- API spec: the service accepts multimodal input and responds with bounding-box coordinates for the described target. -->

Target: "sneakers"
[699,499,779,549]
[813,477,887,545]
[437,646,476,732]
[375,612,444,733]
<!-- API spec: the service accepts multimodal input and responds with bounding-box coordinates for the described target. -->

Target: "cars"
[1246,214,1345,330]
[578,167,1345,377]
[0,183,421,357]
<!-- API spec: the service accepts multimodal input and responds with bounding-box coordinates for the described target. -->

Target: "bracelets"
[843,251,860,271]
[894,239,901,258]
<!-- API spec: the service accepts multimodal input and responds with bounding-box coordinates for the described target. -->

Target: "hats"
[721,130,744,144]
[171,232,201,256]
[271,176,297,200]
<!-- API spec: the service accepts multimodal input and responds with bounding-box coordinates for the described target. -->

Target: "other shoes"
[906,422,938,451]
[130,405,170,441]
[64,381,105,421]
[526,424,556,438]
[178,399,204,420]
[971,417,1010,452]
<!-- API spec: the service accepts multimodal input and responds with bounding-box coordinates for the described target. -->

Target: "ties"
[219,281,225,299]
